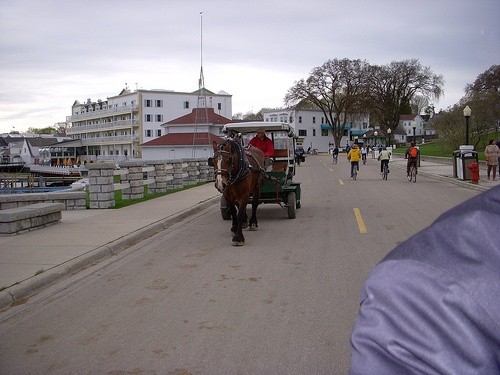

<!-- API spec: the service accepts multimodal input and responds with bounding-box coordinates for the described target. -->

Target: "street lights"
[364,134,366,147]
[462,105,473,144]
[412,120,417,146]
[374,131,378,147]
[387,128,392,148]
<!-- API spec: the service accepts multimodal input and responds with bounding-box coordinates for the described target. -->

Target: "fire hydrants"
[469,162,479,185]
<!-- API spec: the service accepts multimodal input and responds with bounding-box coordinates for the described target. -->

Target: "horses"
[207,132,267,245]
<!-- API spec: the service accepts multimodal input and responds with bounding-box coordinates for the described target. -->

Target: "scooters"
[294,152,303,166]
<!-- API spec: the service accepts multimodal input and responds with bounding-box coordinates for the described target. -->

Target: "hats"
[256,128,265,134]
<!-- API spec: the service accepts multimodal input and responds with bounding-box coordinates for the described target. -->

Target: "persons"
[248,129,274,172]
[378,148,391,175]
[347,185,500,375]
[346,144,350,156]
[360,143,383,162]
[484,139,500,181]
[332,146,339,159]
[296,145,304,157]
[404,143,418,177]
[308,147,311,152]
[347,144,361,180]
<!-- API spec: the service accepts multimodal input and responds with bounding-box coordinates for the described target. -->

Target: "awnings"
[321,124,351,131]
[351,130,367,135]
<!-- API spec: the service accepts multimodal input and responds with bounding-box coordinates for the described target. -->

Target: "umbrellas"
[57,156,91,166]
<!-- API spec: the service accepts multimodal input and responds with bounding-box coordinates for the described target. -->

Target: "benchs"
[270,148,291,171]
[0,202,65,235]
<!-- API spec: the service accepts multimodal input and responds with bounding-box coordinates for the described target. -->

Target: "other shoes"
[487,177,490,180]
[492,178,497,181]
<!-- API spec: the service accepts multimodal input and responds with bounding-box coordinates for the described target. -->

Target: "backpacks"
[409,147,417,158]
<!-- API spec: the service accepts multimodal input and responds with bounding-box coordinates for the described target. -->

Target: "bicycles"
[351,163,358,180]
[333,153,338,164]
[382,161,390,180]
[362,154,367,165]
[404,157,417,183]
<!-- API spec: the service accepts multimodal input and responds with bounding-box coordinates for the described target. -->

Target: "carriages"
[207,121,302,246]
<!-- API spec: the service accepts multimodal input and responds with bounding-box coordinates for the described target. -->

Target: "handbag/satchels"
[485,156,490,161]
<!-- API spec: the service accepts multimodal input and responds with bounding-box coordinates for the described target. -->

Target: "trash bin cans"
[386,147,392,160]
[414,146,420,167]
[372,148,380,159]
[452,150,461,176]
[460,150,479,180]
